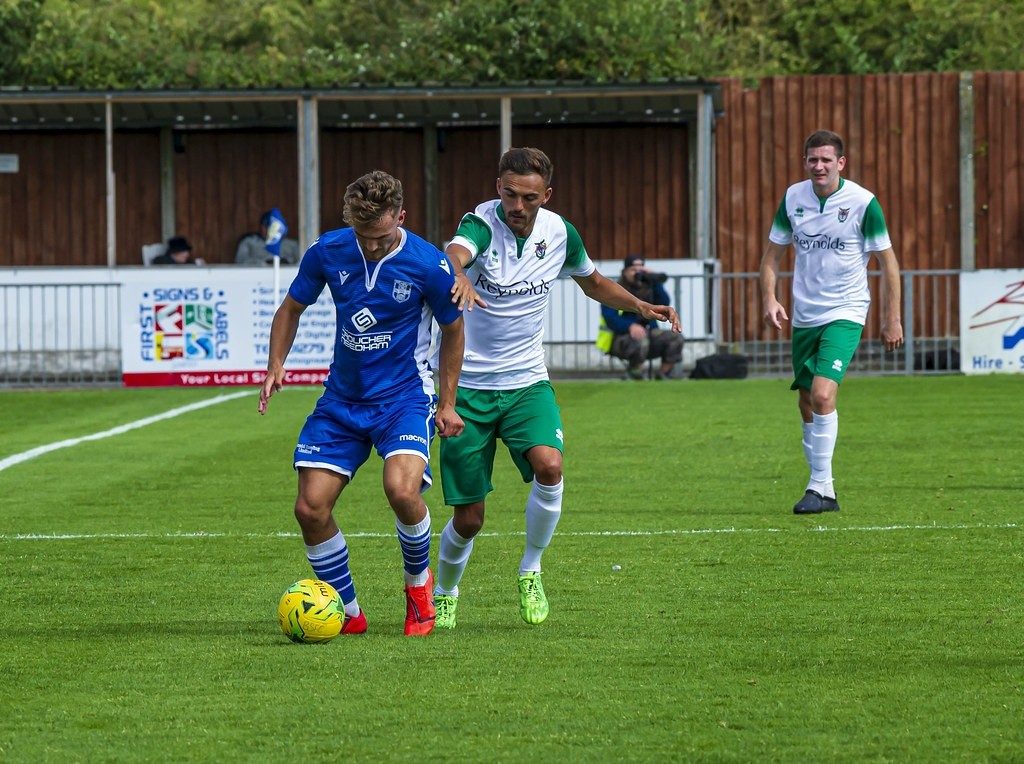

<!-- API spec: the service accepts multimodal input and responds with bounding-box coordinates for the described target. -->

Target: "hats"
[165,235,193,256]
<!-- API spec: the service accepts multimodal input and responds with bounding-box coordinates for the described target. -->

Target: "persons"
[428,146,680,627]
[234,211,299,266]
[758,130,903,514]
[257,166,466,637]
[150,237,196,265]
[599,255,685,381]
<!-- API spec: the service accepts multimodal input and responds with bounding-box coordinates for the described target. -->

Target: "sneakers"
[403,566,437,637]
[339,607,369,635]
[792,487,841,515]
[432,588,460,630]
[516,570,550,625]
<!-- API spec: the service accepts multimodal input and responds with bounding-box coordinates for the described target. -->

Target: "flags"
[265,207,288,257]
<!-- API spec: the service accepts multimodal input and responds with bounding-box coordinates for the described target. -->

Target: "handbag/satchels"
[688,353,750,378]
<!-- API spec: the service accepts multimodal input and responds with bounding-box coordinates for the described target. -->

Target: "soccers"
[276,577,347,645]
